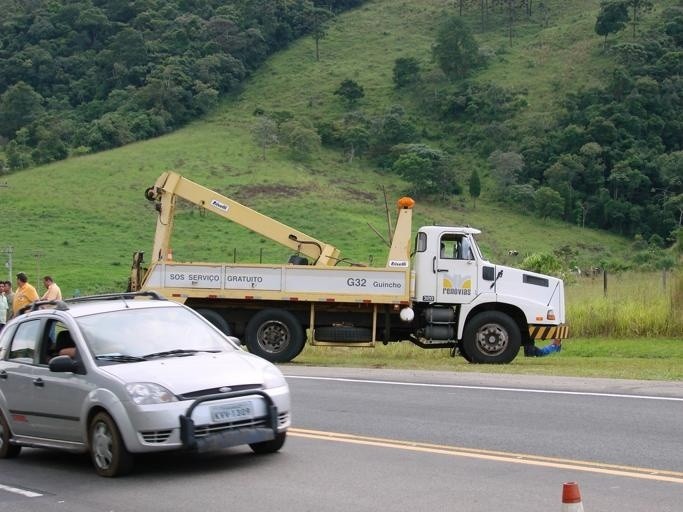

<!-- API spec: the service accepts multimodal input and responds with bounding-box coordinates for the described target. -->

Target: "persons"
[524,338,562,357]
[40,276,62,309]
[0,280,9,332]
[59,337,76,358]
[6,281,15,322]
[12,272,39,317]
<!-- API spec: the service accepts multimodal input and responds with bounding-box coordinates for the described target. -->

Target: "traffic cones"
[560,481,585,512]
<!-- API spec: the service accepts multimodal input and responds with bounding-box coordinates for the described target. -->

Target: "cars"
[0,289,290,478]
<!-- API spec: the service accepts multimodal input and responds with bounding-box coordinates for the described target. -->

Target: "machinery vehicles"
[123,169,570,363]
